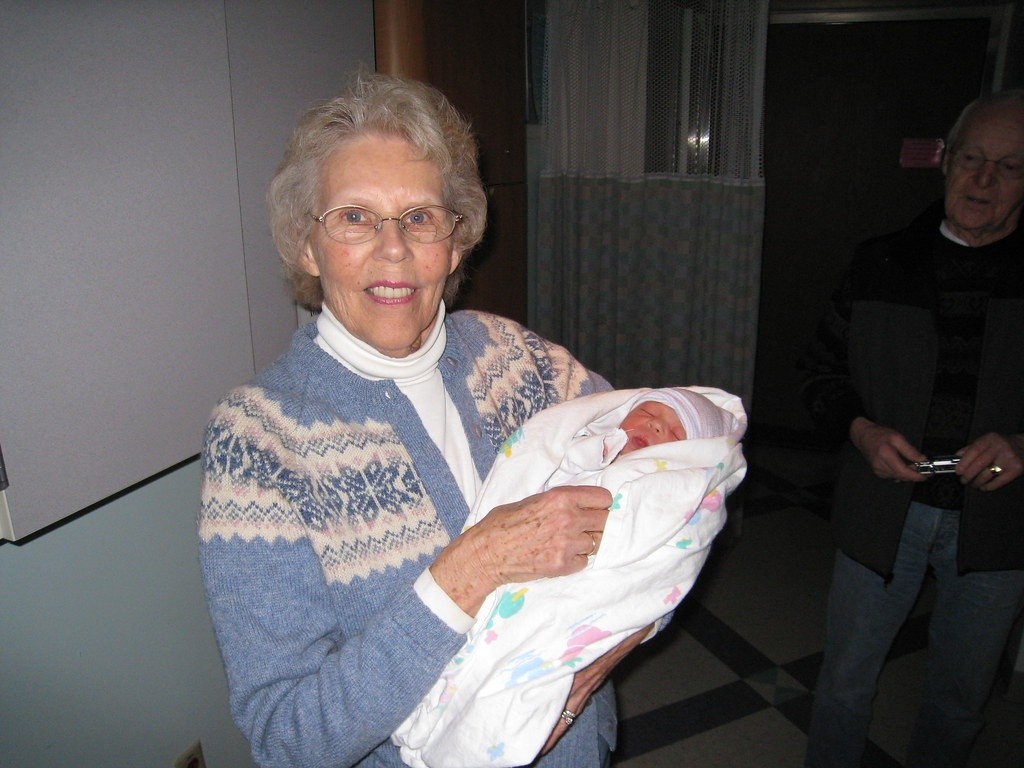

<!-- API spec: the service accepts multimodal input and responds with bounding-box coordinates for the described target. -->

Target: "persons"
[797,87,1023,767]
[197,71,677,767]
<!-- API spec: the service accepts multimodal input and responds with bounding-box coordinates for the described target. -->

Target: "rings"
[578,531,597,556]
[987,463,1001,477]
[561,709,576,725]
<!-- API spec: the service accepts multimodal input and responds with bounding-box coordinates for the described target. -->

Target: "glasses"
[307,203,464,245]
[946,141,1024,179]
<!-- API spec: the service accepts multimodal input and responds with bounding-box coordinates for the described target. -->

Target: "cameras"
[916,455,963,474]
